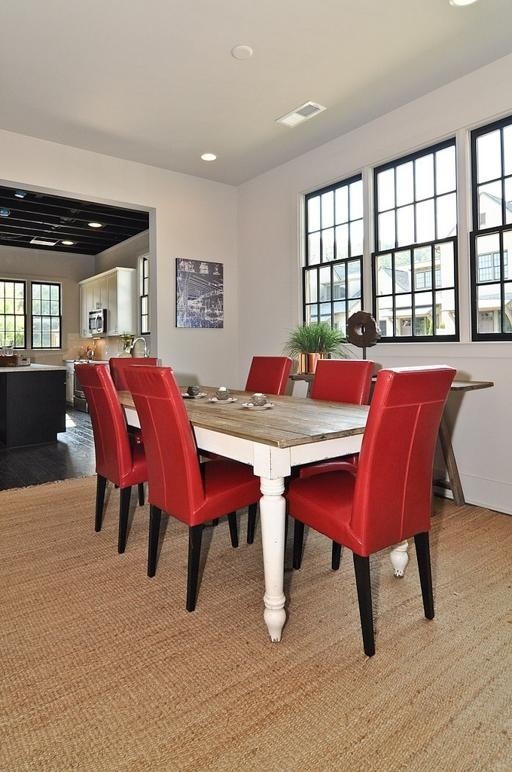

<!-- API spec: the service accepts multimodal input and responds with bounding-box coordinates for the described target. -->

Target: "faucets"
[127,336,152,358]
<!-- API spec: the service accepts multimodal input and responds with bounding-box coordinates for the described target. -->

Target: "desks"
[288,374,495,509]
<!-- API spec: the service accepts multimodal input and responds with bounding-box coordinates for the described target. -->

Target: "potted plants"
[117,329,133,358]
[280,320,358,375]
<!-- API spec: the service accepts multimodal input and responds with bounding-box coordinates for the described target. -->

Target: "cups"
[215,387,231,400]
[250,392,268,407]
[186,385,202,398]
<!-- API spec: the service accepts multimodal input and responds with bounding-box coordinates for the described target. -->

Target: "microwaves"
[87,309,107,335]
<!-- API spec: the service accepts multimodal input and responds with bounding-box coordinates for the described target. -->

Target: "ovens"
[74,361,88,414]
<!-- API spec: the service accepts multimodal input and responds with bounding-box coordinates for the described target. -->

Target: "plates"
[209,397,240,404]
[243,402,276,410]
[181,392,209,400]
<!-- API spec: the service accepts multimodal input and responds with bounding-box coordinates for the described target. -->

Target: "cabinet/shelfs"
[63,361,75,406]
[78,270,137,340]
